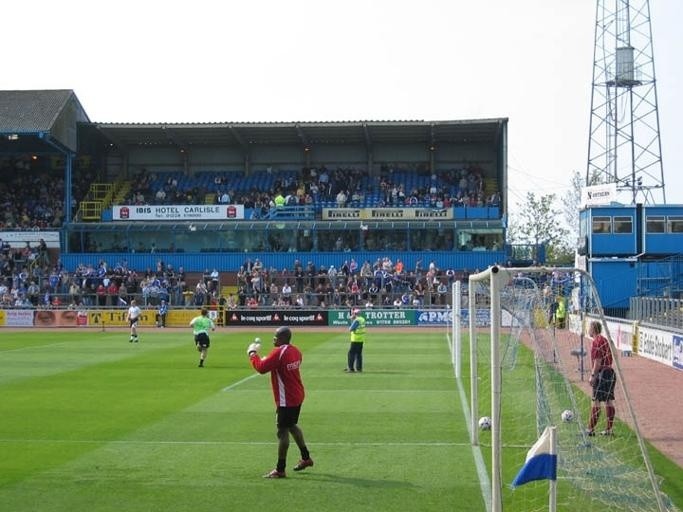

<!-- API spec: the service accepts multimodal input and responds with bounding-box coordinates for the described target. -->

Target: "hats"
[354,309,360,313]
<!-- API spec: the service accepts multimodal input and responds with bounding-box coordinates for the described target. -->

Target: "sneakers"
[129,339,138,342]
[263,469,286,478]
[294,458,313,470]
[345,369,361,372]
[576,431,614,436]
[199,365,204,367]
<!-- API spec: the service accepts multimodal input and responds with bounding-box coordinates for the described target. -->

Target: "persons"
[125,300,142,342]
[0,154,501,309]
[506,260,581,330]
[344,308,365,373]
[585,320,618,437]
[246,324,314,478]
[155,299,169,328]
[189,307,216,367]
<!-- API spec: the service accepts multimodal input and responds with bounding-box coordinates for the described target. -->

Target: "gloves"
[247,343,261,356]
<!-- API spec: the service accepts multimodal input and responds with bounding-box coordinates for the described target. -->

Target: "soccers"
[479,416,492,430]
[255,337,261,344]
[561,410,574,421]
